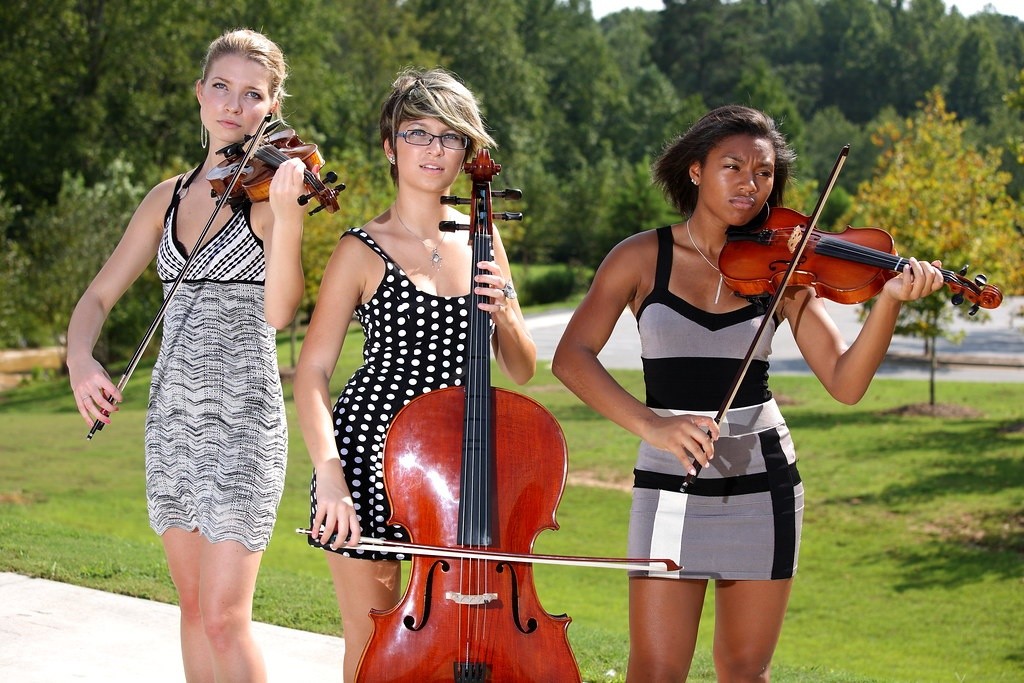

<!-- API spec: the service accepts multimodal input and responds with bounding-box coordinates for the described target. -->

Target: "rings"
[500,279,517,300]
[80,395,90,401]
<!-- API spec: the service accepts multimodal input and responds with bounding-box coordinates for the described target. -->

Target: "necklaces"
[685,217,723,304]
[394,194,452,270]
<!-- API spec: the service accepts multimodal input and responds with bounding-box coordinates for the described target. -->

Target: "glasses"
[395,129,467,149]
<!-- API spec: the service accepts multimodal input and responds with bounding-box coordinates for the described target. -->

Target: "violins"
[204,129,348,216]
[717,206,1003,317]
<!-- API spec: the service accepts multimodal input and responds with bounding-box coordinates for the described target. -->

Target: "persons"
[550,105,943,683]
[66,28,323,683]
[293,63,539,683]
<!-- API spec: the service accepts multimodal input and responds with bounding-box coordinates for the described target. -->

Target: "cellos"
[353,149,582,683]
